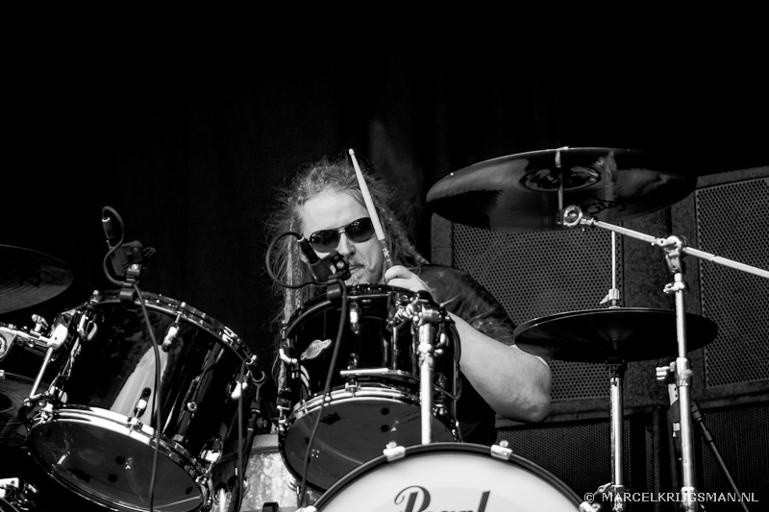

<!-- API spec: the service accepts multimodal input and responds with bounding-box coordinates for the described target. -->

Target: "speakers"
[428,167,769,512]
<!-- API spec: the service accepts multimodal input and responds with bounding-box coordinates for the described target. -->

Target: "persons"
[256,156,555,446]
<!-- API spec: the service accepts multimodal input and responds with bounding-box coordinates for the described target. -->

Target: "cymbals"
[0,244,74,314]
[513,307,717,364]
[427,146,697,233]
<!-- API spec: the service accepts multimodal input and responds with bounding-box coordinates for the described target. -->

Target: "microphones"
[98,215,130,278]
[296,237,334,283]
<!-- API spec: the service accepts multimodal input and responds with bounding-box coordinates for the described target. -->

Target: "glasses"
[307,216,375,254]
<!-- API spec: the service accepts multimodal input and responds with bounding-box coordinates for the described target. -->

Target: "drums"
[211,433,316,512]
[276,282,463,494]
[27,290,252,512]
[292,440,595,512]
